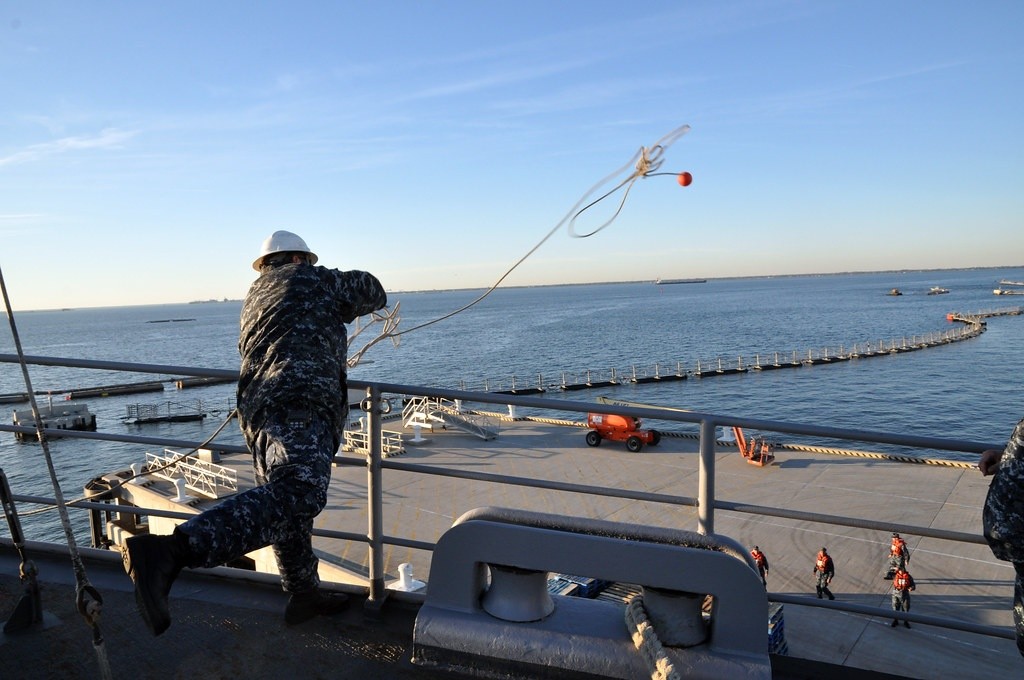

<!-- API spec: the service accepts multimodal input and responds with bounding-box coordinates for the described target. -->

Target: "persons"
[978,417,1024,657]
[883,533,910,580]
[891,564,916,629]
[751,546,769,591]
[123,230,387,638]
[813,548,835,600]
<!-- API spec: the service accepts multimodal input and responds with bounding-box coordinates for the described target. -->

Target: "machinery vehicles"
[584,410,661,453]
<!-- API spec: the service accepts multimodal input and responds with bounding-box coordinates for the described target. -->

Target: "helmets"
[251,229,319,271]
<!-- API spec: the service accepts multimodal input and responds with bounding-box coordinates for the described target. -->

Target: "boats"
[886,289,904,297]
[926,285,951,296]
[12,403,96,441]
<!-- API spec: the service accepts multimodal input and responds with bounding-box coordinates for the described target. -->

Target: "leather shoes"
[285,588,347,624]
[119,534,179,636]
